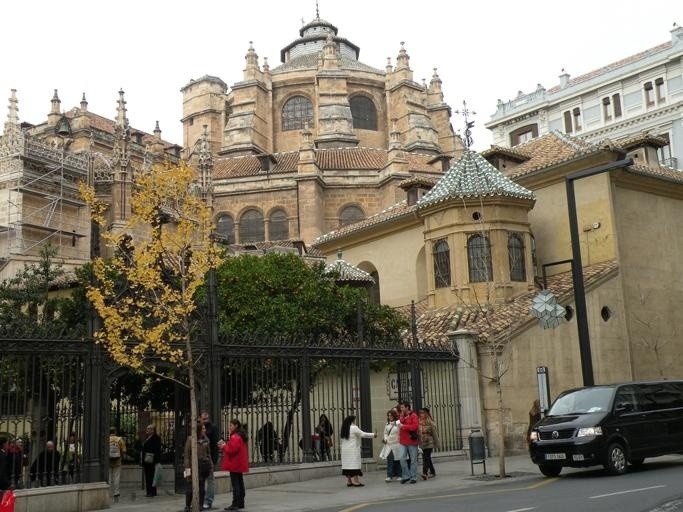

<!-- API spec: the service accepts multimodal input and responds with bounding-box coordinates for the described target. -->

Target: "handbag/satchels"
[145,452,155,462]
[199,458,212,474]
[382,424,394,444]
[404,411,418,441]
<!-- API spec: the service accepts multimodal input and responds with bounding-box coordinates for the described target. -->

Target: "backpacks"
[109,436,122,459]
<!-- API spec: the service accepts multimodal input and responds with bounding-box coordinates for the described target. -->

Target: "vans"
[525,378,682,476]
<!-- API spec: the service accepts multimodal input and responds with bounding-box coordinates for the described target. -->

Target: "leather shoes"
[400,479,409,484]
[346,484,353,486]
[410,480,416,484]
[355,483,365,487]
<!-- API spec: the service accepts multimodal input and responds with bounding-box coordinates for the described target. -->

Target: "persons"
[66,432,82,482]
[418,408,440,480]
[315,414,333,463]
[526,399,542,443]
[197,410,219,509]
[256,422,279,463]
[12,437,29,489]
[391,406,401,419]
[397,400,419,485]
[340,416,377,486]
[30,440,60,488]
[379,409,409,483]
[0,434,15,512]
[217,419,250,511]
[299,435,321,461]
[141,424,161,497]
[182,422,211,512]
[109,426,127,497]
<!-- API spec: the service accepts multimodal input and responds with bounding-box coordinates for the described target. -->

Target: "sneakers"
[225,505,240,511]
[384,478,393,481]
[420,474,427,480]
[203,504,210,509]
[396,477,402,480]
[428,473,435,478]
